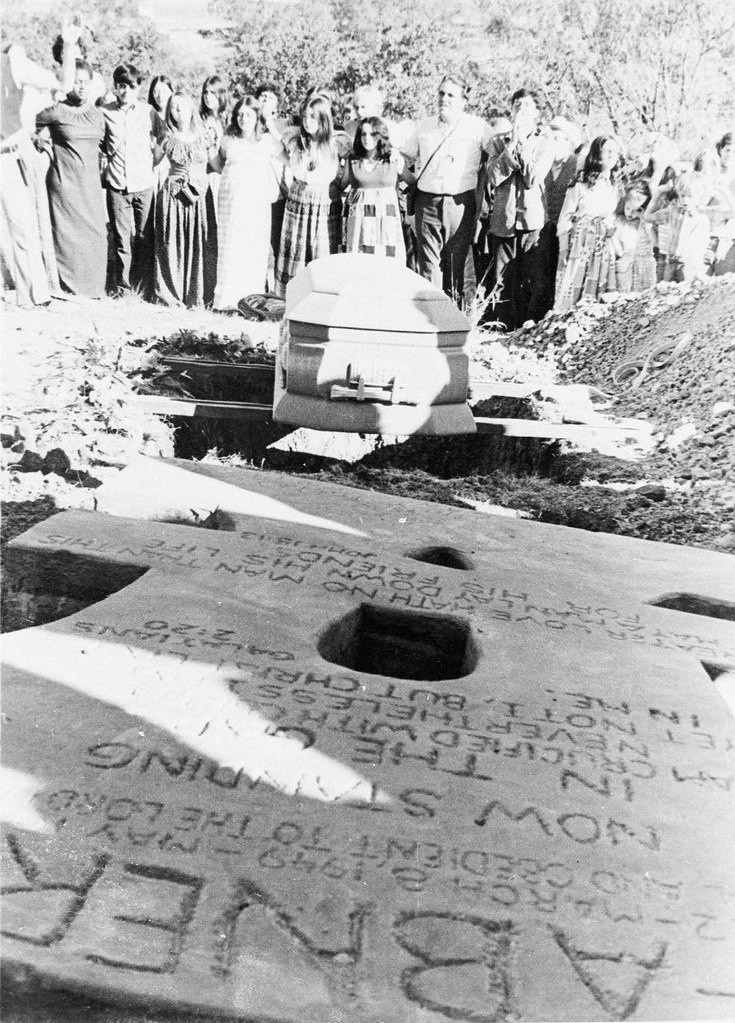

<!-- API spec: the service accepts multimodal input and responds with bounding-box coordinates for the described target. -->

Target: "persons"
[0,25,731,317]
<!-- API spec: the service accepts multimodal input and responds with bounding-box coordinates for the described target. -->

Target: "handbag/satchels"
[401,179,416,217]
